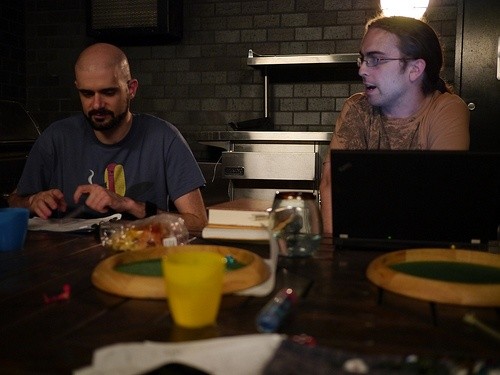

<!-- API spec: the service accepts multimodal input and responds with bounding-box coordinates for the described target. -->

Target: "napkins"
[28,213,122,232]
[73,333,288,375]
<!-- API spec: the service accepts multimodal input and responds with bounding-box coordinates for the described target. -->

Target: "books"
[28,213,121,231]
[207,198,273,228]
[201,224,275,240]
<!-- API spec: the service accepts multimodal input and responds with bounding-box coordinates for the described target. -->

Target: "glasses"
[356,54,421,68]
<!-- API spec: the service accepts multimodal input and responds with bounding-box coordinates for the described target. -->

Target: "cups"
[162,252,225,328]
[268,198,324,257]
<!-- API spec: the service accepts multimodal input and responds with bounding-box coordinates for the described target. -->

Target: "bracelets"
[144,202,157,218]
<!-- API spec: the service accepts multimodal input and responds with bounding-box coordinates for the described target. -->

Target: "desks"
[0,221,500,375]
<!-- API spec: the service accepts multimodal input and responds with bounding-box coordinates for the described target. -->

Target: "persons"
[9,42,210,232]
[320,17,471,235]
